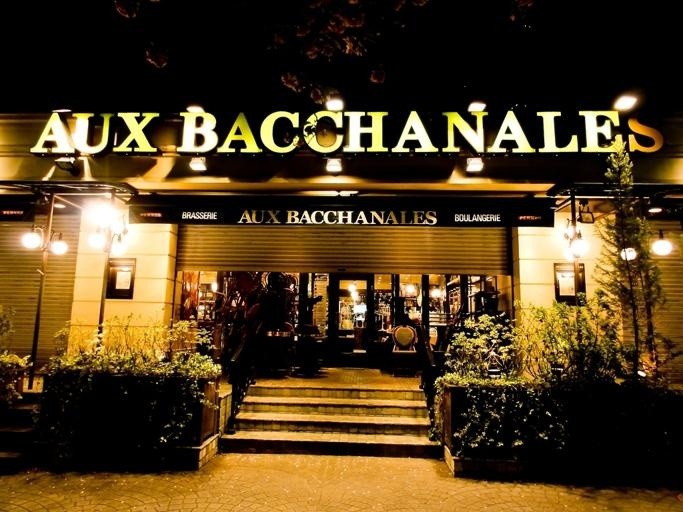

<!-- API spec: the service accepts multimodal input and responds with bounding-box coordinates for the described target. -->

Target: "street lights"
[564,216,588,374]
[20,221,68,389]
[619,228,671,383]
[84,200,129,359]
